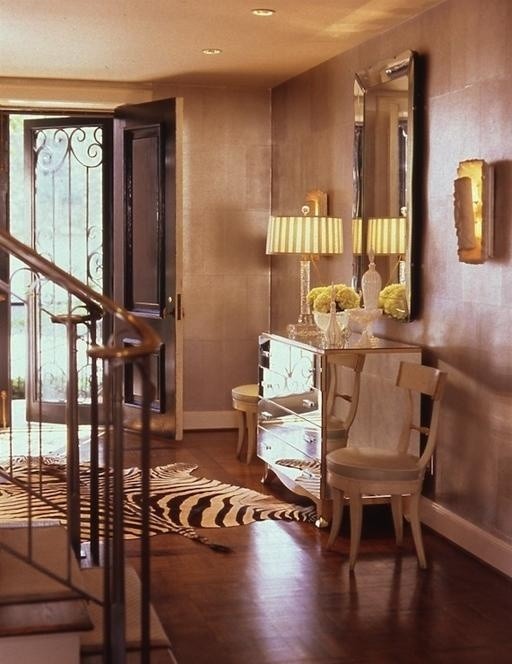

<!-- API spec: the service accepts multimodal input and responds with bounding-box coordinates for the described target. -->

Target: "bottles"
[324,302,342,345]
[360,255,382,309]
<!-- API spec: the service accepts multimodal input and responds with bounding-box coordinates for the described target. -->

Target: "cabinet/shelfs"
[256,328,423,528]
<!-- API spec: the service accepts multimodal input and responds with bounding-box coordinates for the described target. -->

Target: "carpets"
[0,454,316,553]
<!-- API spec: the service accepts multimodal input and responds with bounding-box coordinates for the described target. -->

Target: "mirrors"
[351,48,420,323]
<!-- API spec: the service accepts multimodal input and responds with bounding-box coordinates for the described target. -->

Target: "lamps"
[351,217,406,254]
[265,216,343,340]
[453,158,495,264]
[301,189,328,216]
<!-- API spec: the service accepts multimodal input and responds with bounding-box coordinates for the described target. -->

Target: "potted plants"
[305,283,361,331]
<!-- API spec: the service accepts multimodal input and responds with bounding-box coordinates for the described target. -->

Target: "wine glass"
[345,308,383,348]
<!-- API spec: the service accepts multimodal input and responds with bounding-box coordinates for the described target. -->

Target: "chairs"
[325,360,448,571]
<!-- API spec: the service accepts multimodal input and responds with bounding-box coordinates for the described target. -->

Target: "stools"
[232,383,259,464]
